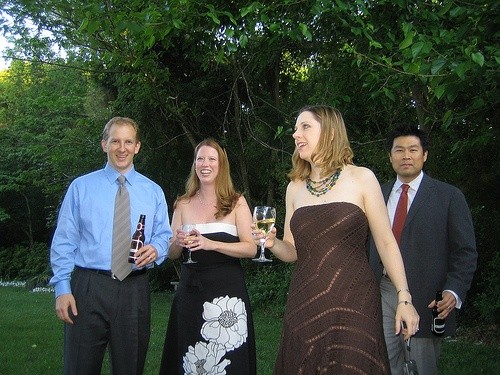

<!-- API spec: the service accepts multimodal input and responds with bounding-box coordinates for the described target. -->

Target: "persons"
[250,105,420,374]
[368,124,478,375]
[158,138,258,375]
[48,117,174,375]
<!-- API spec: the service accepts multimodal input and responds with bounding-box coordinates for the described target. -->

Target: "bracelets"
[397,289,410,294]
[398,301,413,305]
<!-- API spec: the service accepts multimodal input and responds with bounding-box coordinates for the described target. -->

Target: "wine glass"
[183,224,197,265]
[251,205,276,263]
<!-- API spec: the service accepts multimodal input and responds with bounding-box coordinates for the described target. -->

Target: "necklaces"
[197,189,215,206]
[305,169,341,197]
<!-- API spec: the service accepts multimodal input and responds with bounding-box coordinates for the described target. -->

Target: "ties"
[392,184,409,246]
[112,175,132,281]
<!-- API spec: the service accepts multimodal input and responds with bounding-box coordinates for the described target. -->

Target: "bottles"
[128,215,146,264]
[429,290,446,336]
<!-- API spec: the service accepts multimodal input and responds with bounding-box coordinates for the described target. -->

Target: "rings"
[448,306,451,310]
[148,256,152,261]
